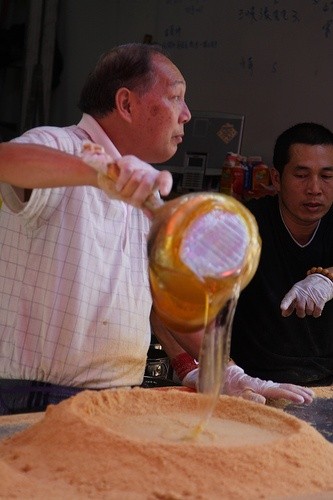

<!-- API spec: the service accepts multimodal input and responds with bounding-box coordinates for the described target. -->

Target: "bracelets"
[308,267,333,281]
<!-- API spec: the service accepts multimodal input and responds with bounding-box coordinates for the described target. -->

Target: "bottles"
[219,152,270,201]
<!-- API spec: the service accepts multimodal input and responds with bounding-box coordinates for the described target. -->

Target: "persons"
[0,43,315,416]
[227,121,333,388]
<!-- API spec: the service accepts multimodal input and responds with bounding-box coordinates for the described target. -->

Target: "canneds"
[252,164,268,191]
[220,166,244,201]
